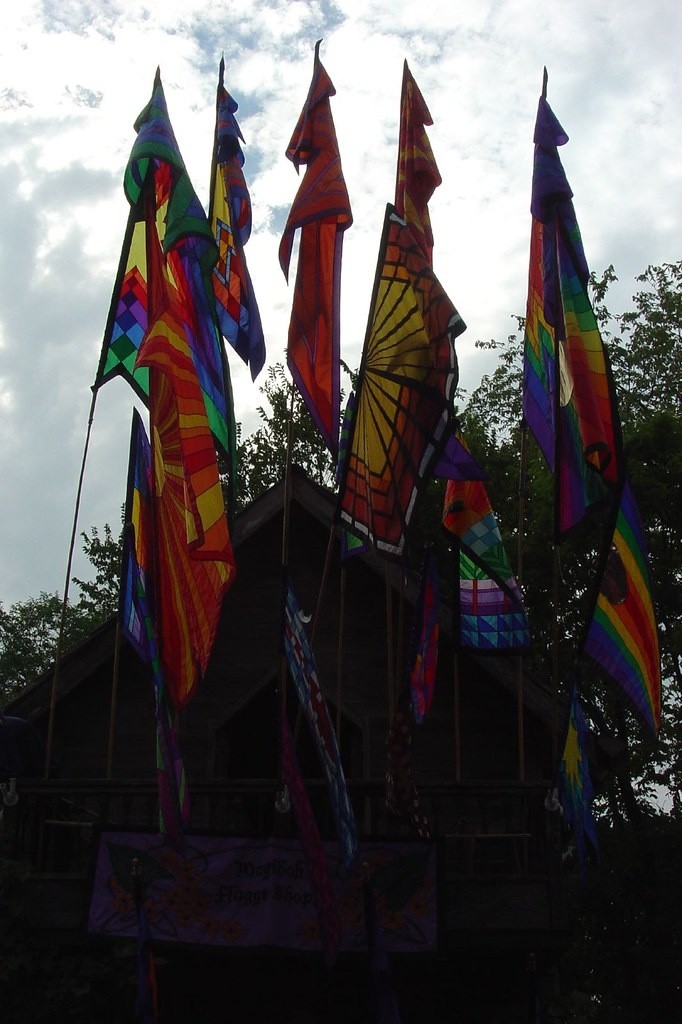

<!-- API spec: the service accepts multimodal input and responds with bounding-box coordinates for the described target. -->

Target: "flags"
[92,39,662,1024]
